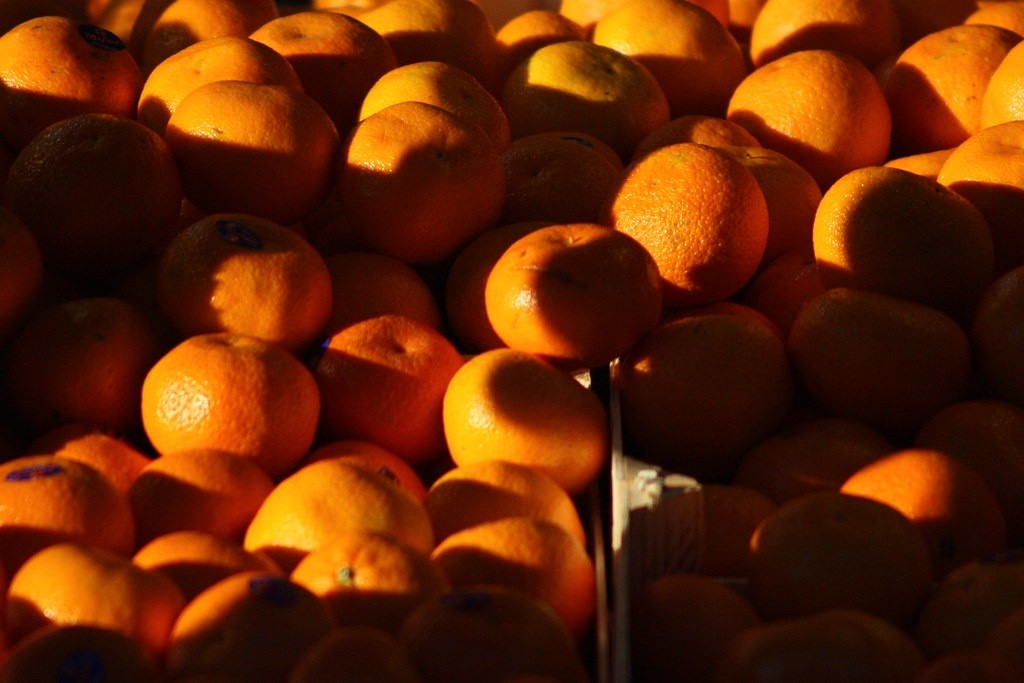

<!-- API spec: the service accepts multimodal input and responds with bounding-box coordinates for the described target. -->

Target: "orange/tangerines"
[0,0,1024,683]
